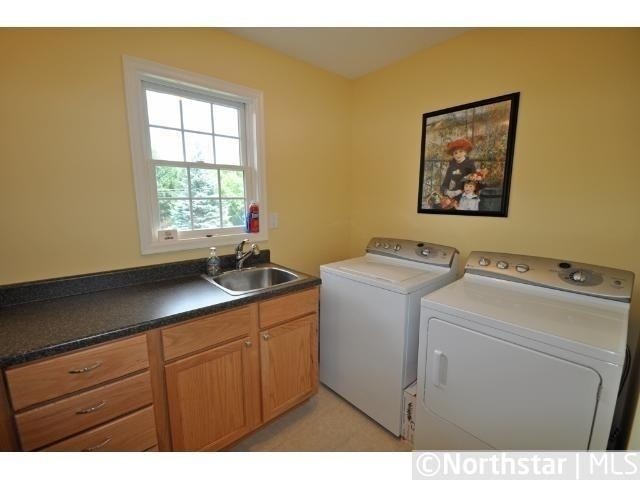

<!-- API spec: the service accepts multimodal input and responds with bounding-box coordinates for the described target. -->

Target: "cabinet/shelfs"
[3,331,161,451]
[159,284,319,452]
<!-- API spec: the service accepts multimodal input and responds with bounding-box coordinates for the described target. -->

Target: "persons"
[435,136,484,210]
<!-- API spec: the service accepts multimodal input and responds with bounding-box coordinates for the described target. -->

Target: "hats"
[446,138,473,156]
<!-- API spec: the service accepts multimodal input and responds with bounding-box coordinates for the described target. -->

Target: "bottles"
[208,247,220,276]
[247,201,260,233]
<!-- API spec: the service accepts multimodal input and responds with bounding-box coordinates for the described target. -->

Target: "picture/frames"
[416,91,520,217]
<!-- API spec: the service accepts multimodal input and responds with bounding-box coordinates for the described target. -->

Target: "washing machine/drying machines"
[319,236,461,439]
[411,250,635,451]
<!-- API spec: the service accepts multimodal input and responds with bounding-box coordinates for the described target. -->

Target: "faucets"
[235,239,261,269]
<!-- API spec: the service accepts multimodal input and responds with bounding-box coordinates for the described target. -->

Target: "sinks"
[212,264,300,294]
